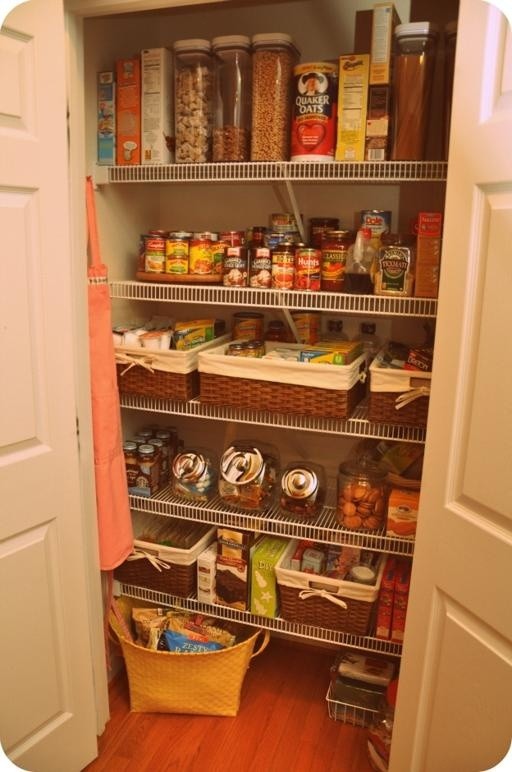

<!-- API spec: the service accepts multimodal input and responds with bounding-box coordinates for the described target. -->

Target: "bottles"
[264,320,287,341]
[279,461,327,522]
[321,229,354,292]
[389,20,439,162]
[242,342,258,358]
[130,435,146,444]
[173,38,212,164]
[336,461,389,531]
[294,242,321,291]
[270,213,304,243]
[148,439,163,477]
[250,32,303,162]
[353,322,381,348]
[249,339,265,358]
[156,430,173,476]
[309,217,340,249]
[322,318,348,340]
[123,441,137,487]
[169,447,219,502]
[211,34,250,162]
[137,428,153,443]
[217,439,281,510]
[270,240,294,290]
[360,210,392,252]
[226,343,248,357]
[442,20,457,161]
[137,444,160,494]
[373,231,417,297]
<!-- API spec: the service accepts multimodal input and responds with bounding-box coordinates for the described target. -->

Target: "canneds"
[288,311,322,346]
[249,246,271,289]
[223,246,248,287]
[194,232,218,241]
[168,231,193,237]
[289,61,339,162]
[145,238,166,273]
[165,236,192,275]
[219,231,247,248]
[231,312,264,341]
[212,240,231,274]
[188,239,212,274]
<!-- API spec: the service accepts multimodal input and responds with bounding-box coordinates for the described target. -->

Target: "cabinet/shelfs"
[85,1,459,659]
